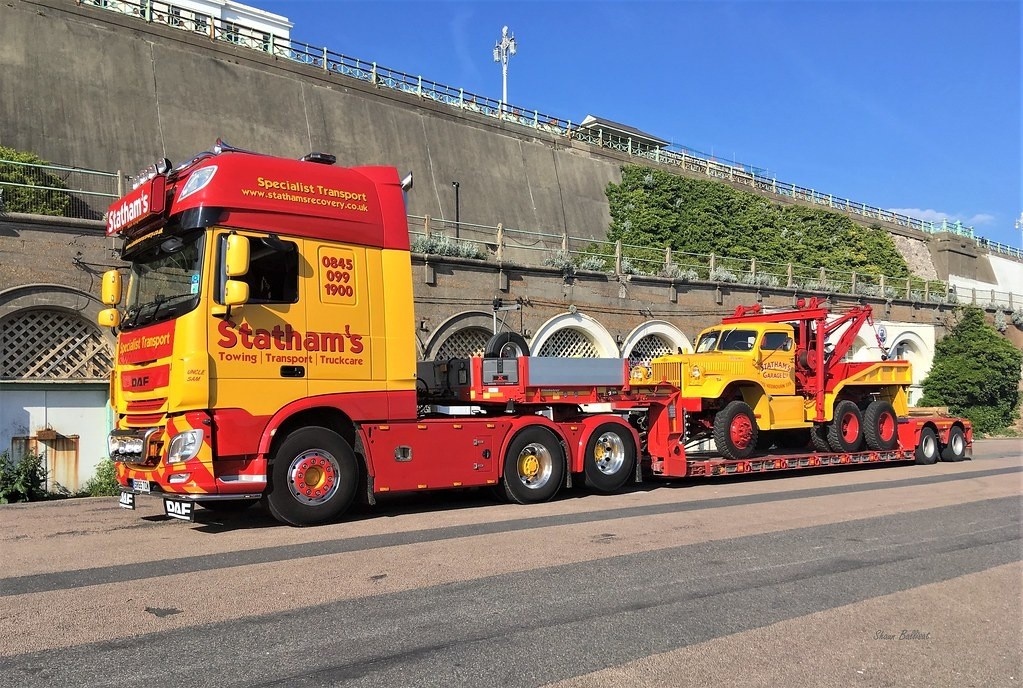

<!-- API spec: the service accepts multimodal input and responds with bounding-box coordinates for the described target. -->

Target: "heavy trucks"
[648,297,913,461]
[97,136,977,530]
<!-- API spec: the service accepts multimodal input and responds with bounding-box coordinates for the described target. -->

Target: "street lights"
[451,181,460,243]
